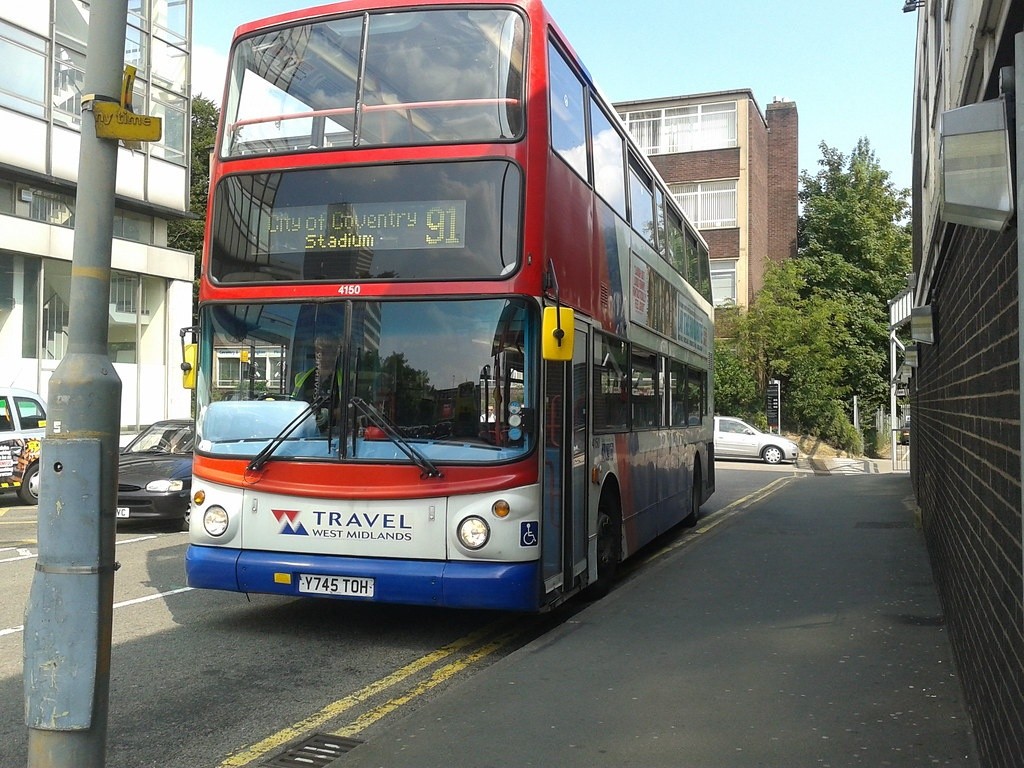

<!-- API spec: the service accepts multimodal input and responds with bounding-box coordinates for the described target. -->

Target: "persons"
[480,404,499,423]
[292,332,360,435]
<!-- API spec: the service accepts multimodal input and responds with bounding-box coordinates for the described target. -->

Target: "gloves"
[317,408,328,426]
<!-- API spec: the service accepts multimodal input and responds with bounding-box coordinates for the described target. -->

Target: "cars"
[681,415,799,464]
[900,415,910,445]
[117,420,195,531]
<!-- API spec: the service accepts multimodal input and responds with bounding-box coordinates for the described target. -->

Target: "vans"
[0,385,47,505]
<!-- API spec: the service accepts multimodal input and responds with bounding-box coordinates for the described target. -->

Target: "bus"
[179,0,715,615]
